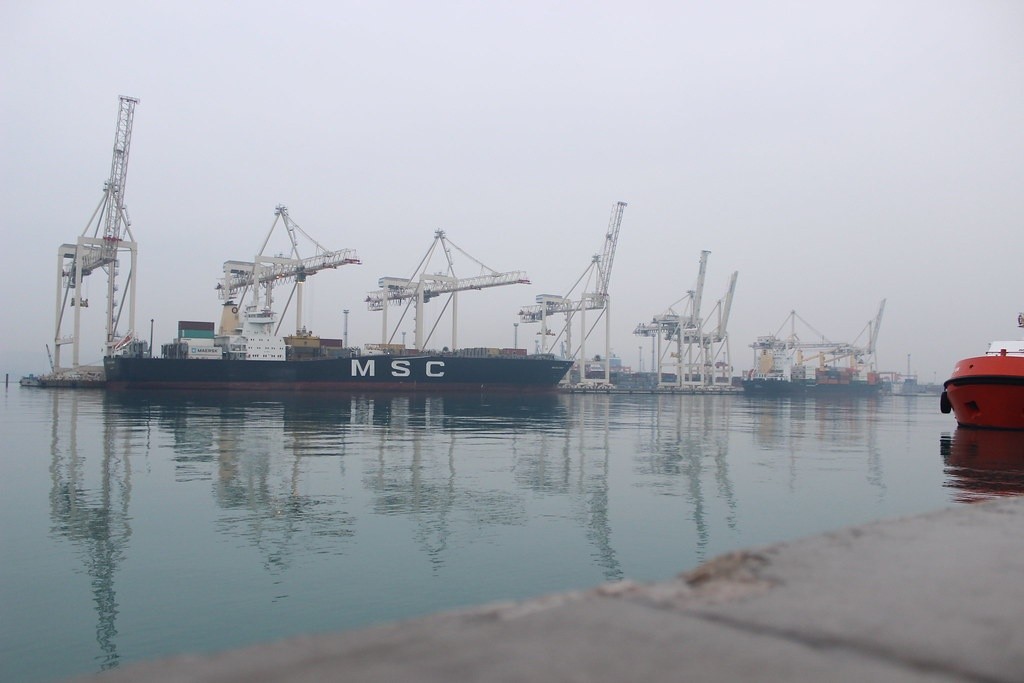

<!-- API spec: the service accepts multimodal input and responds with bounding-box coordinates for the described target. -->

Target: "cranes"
[635,249,886,389]
[216,204,363,336]
[367,227,531,352]
[53,96,142,375]
[519,200,628,388]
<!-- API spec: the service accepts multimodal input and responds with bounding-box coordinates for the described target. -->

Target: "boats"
[741,347,885,399]
[941,423,1024,505]
[103,262,575,400]
[940,309,1024,430]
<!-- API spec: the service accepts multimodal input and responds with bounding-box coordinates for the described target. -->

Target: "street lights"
[343,309,350,349]
[514,323,519,349]
